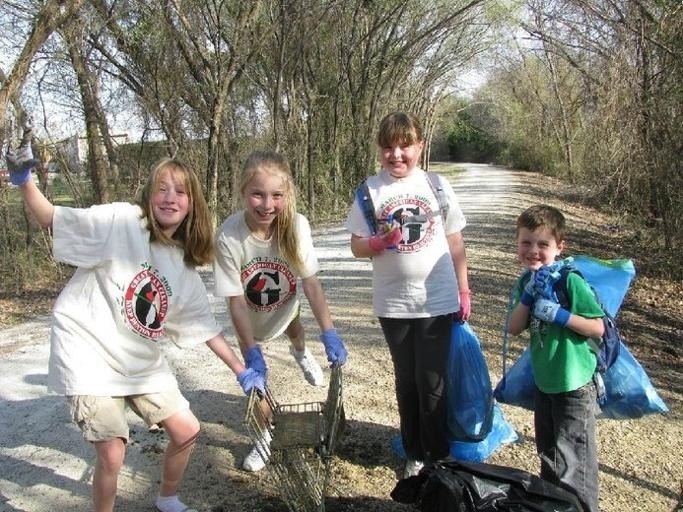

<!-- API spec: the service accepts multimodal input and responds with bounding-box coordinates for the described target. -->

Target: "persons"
[213,152,347,469]
[506,205,609,511]
[347,111,473,477]
[4,122,264,512]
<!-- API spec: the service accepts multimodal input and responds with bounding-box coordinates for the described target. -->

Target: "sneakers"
[242,418,276,472]
[156,492,197,512]
[404,459,425,479]
[288,345,324,386]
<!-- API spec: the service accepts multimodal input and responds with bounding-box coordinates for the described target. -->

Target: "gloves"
[457,289,471,321]
[234,342,269,398]
[3,123,42,186]
[532,297,572,328]
[368,222,405,258]
[319,328,348,370]
[520,264,559,307]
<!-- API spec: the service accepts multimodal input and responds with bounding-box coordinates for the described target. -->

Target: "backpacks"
[522,265,621,374]
